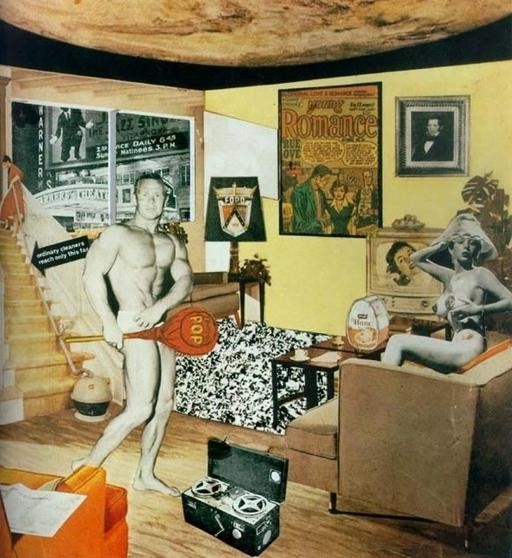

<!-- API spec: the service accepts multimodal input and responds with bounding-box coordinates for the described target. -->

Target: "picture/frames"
[395,95,470,176]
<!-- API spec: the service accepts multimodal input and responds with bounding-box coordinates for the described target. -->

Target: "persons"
[323,181,356,236]
[48,107,96,161]
[351,168,380,237]
[289,163,334,236]
[383,239,434,288]
[70,172,195,499]
[0,154,27,234]
[410,114,455,161]
[380,212,512,373]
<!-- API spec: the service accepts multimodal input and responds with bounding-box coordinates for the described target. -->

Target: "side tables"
[227,274,268,328]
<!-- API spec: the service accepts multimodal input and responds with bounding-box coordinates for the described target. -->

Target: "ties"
[67,113,70,120]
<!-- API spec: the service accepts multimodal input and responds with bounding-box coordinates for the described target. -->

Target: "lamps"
[203,176,267,280]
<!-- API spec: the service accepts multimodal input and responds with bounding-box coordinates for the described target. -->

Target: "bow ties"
[426,135,436,141]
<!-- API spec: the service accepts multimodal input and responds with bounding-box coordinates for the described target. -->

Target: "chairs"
[0,459,132,557]
[159,270,243,336]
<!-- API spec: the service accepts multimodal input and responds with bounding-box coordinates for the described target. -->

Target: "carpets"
[159,322,347,441]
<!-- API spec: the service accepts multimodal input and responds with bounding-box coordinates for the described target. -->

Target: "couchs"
[280,324,510,554]
